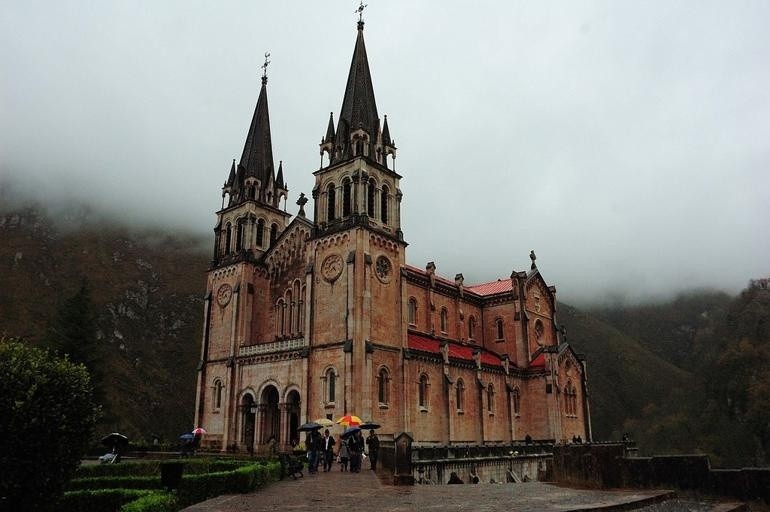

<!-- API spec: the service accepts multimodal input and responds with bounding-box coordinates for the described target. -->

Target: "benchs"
[276,451,304,481]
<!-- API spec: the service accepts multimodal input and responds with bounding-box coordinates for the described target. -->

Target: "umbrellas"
[359,422,381,430]
[336,415,362,426]
[101,433,128,444]
[314,418,334,427]
[297,422,323,432]
[192,428,206,434]
[180,434,196,440]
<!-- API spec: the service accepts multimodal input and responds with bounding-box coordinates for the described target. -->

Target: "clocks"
[215,283,232,308]
[320,252,343,282]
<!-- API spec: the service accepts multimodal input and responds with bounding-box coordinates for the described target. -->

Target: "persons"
[304,427,380,475]
